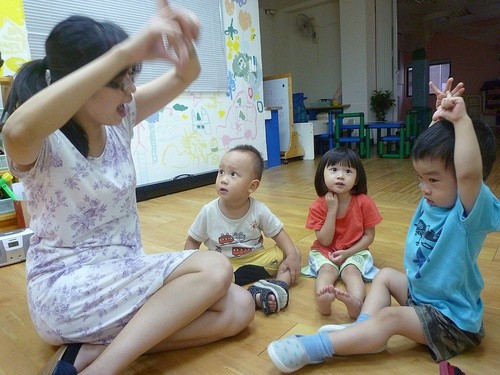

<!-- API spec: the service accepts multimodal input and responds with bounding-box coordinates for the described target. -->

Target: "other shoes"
[38,342,84,375]
[439,361,466,375]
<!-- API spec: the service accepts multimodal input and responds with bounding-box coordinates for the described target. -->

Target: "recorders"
[0,227,33,267]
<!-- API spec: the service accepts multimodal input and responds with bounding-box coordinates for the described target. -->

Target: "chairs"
[335,106,432,163]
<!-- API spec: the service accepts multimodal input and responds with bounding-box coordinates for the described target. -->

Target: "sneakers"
[318,320,361,335]
[267,334,325,373]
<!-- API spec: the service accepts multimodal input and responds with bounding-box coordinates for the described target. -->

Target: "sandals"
[247,278,289,316]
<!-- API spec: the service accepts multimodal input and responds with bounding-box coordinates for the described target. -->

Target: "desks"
[357,119,406,158]
[304,104,351,154]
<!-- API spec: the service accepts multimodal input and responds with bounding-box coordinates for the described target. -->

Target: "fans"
[297,14,320,41]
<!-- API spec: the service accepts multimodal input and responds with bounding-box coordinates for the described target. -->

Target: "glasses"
[108,61,142,94]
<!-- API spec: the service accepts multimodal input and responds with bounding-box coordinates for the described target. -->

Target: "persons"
[0,0,256,375]
[300,148,382,319]
[267,77,500,372]
[184,144,302,314]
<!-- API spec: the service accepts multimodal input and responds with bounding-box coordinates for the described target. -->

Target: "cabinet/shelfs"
[465,95,482,116]
[0,75,26,231]
[481,89,500,126]
